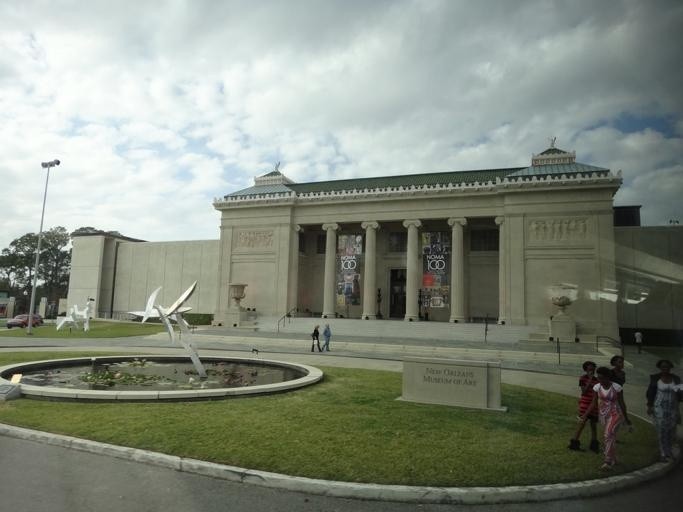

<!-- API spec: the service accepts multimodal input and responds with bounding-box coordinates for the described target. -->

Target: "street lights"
[668,220,679,226]
[27,158,60,334]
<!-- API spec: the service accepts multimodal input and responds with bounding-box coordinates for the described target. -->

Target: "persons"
[310,325,321,352]
[568,362,600,454]
[632,329,642,353]
[321,324,330,352]
[577,367,632,472]
[644,360,682,462]
[608,356,631,430]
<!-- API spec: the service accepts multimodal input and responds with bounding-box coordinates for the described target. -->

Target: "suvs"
[7,314,42,329]
